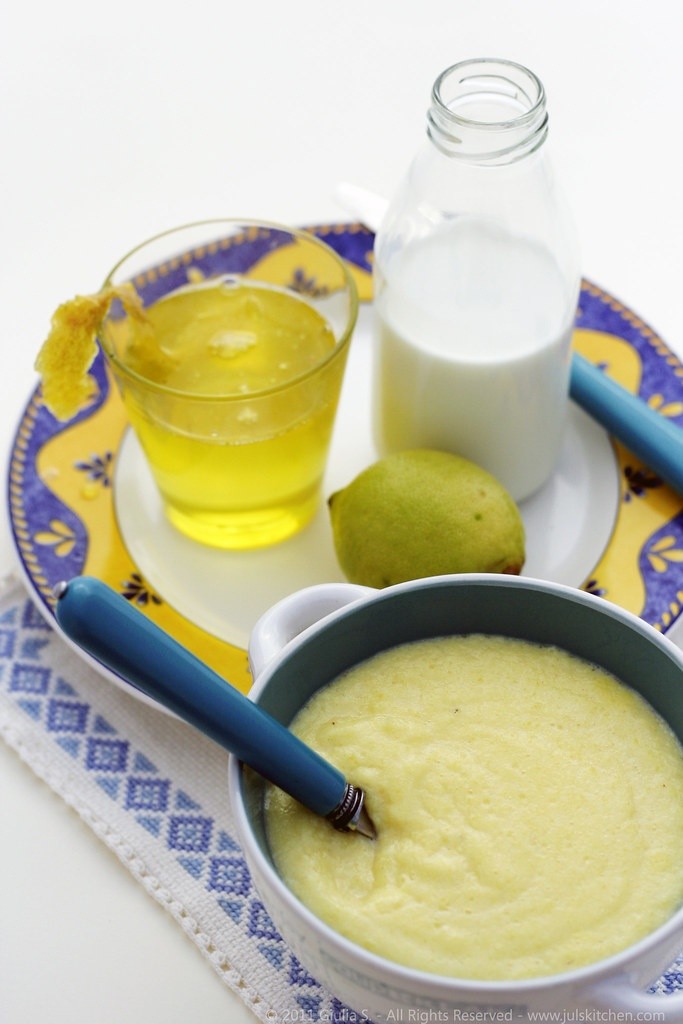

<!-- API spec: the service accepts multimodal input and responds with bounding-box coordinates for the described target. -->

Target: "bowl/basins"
[224,571,682,1024]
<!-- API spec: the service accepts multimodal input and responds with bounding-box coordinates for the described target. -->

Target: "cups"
[96,222,360,550]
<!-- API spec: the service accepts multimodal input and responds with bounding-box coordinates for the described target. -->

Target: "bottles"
[371,60,579,505]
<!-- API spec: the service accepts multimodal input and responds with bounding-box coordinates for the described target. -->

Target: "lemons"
[325,446,526,589]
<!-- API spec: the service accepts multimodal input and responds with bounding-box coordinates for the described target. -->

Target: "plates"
[6,222,683,728]
[115,305,623,646]
